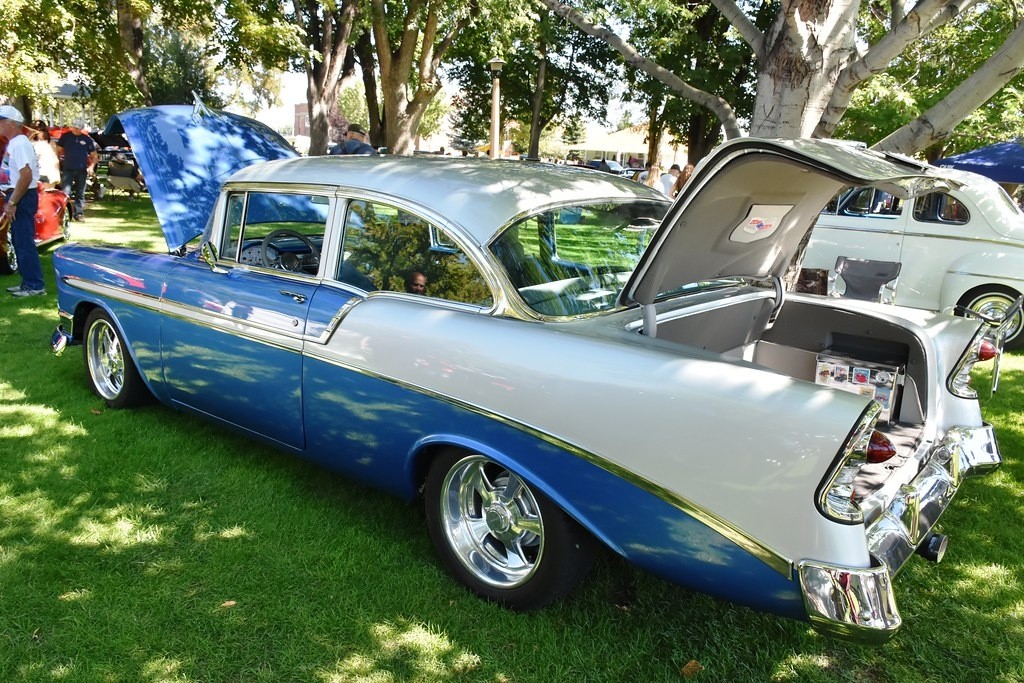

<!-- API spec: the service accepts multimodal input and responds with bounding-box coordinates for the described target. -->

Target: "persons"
[58,120,98,225]
[404,272,424,295]
[108,153,143,183]
[0,106,64,300]
[639,159,695,198]
[328,124,377,154]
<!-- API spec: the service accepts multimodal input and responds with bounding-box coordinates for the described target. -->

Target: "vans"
[590,160,623,174]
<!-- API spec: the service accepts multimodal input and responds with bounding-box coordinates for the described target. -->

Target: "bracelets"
[9,200,17,206]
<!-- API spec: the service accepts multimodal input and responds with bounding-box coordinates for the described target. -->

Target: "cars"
[49,101,1001,646]
[0,121,99,275]
[732,160,1024,350]
[616,168,645,180]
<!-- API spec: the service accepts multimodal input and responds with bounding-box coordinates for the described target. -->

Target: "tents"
[929,137,1024,185]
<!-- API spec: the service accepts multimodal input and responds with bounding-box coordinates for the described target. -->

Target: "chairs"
[107,175,139,201]
[828,255,903,304]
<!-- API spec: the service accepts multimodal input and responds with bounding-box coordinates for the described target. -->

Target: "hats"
[343,124,365,136]
[671,164,682,173]
[0,105,23,123]
[72,117,85,129]
[116,153,127,162]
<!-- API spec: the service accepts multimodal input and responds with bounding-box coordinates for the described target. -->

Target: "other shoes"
[75,214,85,223]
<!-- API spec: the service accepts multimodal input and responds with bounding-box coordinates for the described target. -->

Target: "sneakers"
[12,289,48,297]
[7,285,20,291]
